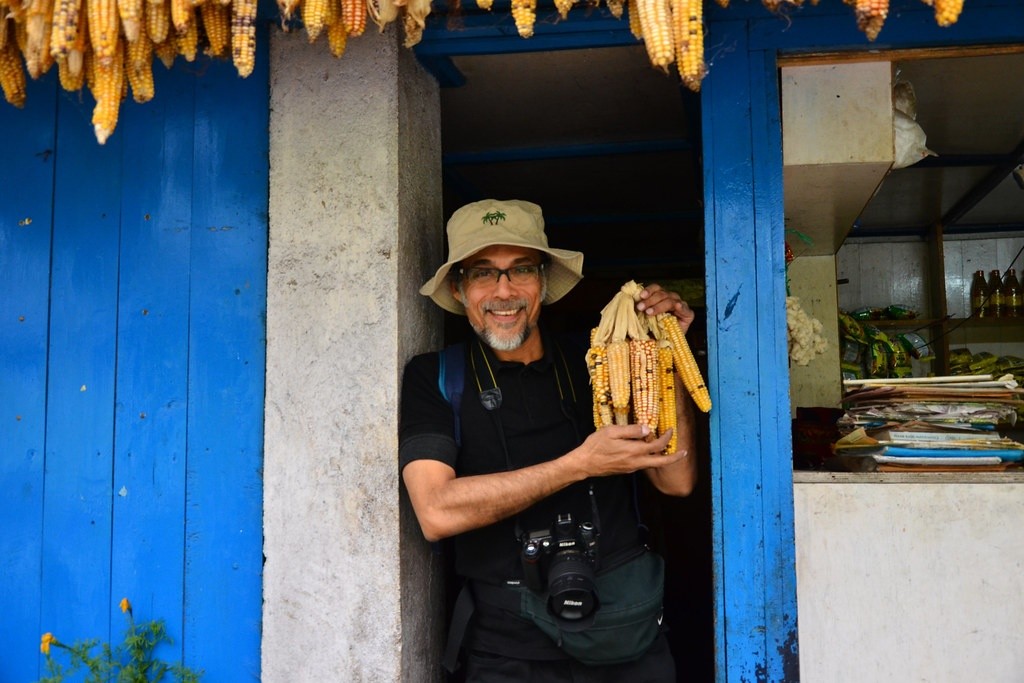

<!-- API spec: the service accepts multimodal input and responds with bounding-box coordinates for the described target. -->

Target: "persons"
[398,199,699,683]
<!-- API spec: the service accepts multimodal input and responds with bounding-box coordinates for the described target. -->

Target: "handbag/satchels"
[520,540,681,665]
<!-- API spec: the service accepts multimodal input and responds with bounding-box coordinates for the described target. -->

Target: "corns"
[0,0,972,145]
[584,280,712,456]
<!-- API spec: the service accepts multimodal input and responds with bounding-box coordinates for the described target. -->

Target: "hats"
[418,199,584,315]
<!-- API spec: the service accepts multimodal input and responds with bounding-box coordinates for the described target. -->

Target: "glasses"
[459,263,544,288]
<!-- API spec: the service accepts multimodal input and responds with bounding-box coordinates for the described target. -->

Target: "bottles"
[971,268,1024,319]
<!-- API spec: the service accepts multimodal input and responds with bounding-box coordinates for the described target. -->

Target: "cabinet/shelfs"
[835,227,1023,376]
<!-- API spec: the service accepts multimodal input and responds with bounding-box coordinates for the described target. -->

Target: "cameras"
[516,512,604,634]
